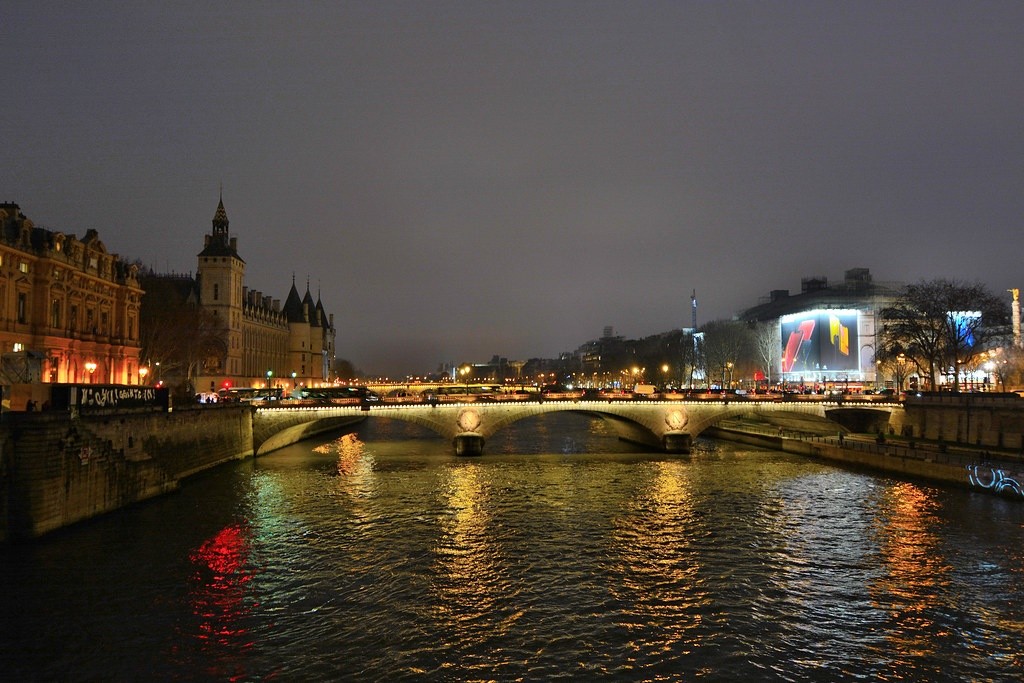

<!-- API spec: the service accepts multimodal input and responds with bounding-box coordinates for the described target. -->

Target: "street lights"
[292,373,297,389]
[267,371,273,404]
[226,382,229,402]
[461,366,471,396]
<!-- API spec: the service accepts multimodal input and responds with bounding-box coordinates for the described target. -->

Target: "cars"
[387,389,416,397]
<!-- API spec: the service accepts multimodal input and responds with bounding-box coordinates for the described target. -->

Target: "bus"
[298,387,365,403]
[218,386,284,402]
